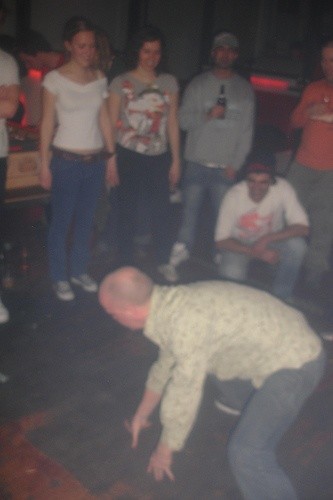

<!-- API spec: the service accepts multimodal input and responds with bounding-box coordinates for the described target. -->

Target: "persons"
[161,31,256,284]
[283,36,333,300]
[98,265,326,500]
[40,18,120,301]
[0,47,20,322]
[106,27,181,270]
[211,154,310,305]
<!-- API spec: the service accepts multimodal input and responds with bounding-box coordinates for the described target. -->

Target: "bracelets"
[105,148,115,161]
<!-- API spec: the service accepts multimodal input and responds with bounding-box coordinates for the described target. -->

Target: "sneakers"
[70,274,98,292]
[54,281,76,301]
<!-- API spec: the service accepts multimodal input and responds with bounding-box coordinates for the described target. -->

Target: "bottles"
[217,85,226,119]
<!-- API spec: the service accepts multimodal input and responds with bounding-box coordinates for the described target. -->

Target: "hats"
[213,33,239,49]
[245,154,276,186]
